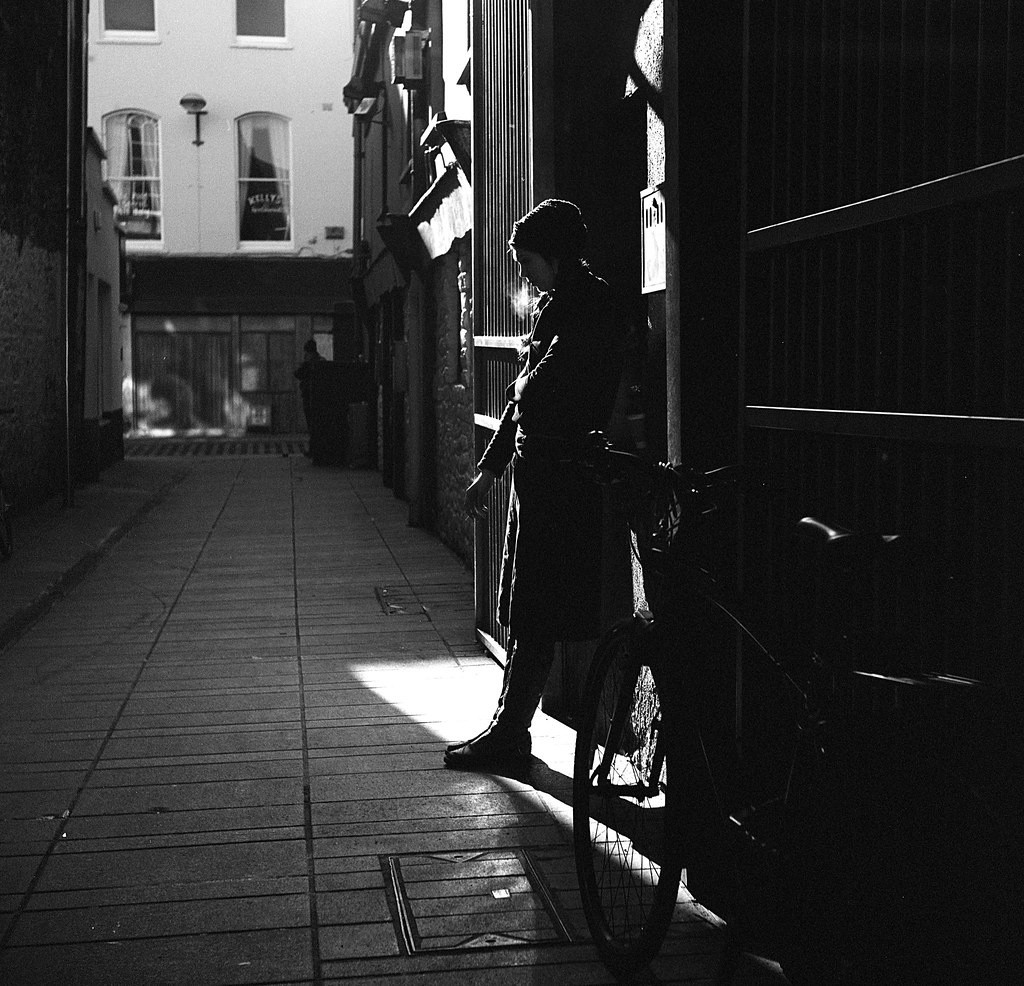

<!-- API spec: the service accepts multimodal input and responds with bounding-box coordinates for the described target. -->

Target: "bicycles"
[527,429,1023,985]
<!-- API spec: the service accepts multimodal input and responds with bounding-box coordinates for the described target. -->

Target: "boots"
[443,638,556,779]
[445,638,516,755]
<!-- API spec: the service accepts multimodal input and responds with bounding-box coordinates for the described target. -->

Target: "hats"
[507,198,588,260]
[304,339,317,352]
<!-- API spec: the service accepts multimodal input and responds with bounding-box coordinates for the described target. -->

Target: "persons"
[294,339,332,455]
[442,199,637,773]
